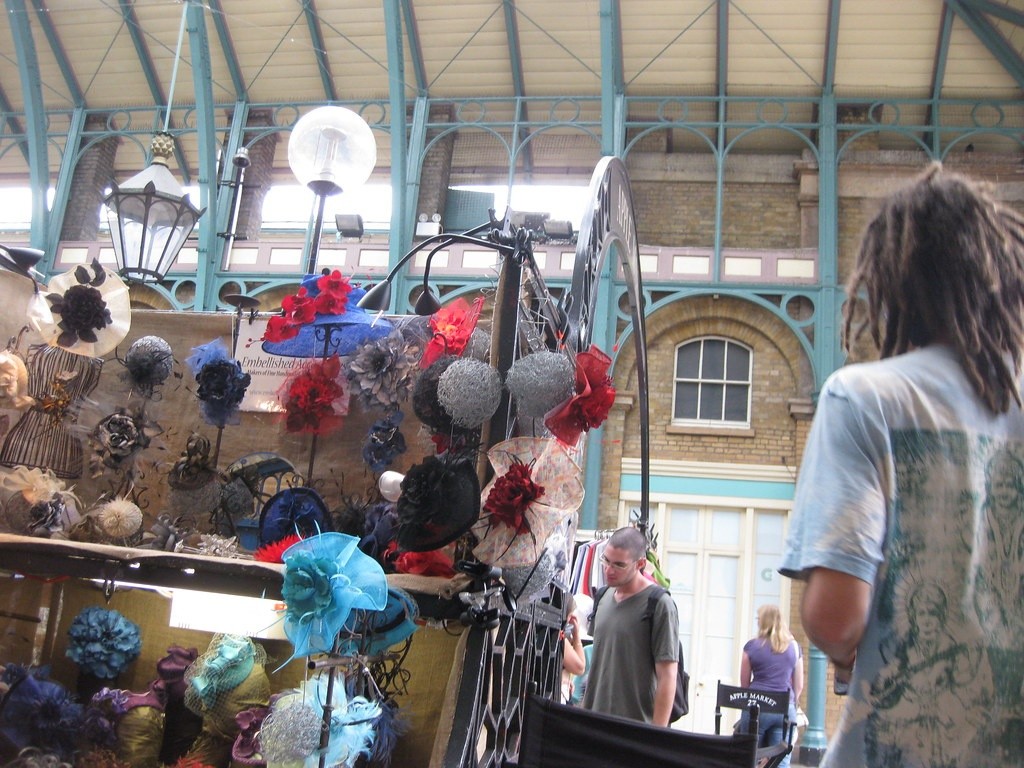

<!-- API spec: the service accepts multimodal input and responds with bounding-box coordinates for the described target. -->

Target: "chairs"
[714,679,797,768]
[516,681,760,768]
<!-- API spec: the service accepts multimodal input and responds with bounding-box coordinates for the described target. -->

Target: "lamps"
[336,214,366,238]
[415,212,443,242]
[99,0,209,283]
[541,222,574,240]
[0,244,46,272]
[356,222,515,316]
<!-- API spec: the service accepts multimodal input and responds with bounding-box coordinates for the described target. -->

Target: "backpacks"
[586,585,690,724]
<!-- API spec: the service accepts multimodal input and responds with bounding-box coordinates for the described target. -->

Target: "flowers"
[0,261,618,768]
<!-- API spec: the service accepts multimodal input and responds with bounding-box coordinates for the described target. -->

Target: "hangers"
[590,529,612,542]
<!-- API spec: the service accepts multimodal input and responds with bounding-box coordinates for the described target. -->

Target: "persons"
[582,527,679,727]
[176,633,270,768]
[559,592,586,704]
[233,673,377,768]
[85,688,164,768]
[739,603,803,768]
[778,164,1024,768]
